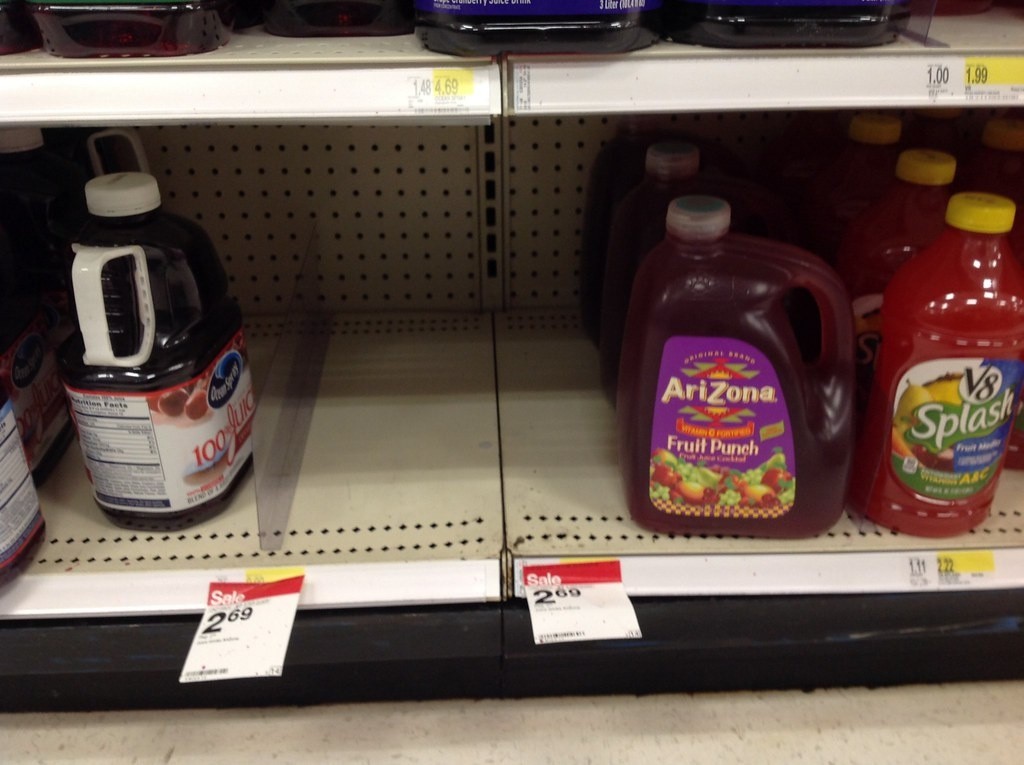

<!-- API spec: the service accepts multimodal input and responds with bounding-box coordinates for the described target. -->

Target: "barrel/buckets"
[0,125,256,592]
[588,109,1024,536]
[0,0,922,58]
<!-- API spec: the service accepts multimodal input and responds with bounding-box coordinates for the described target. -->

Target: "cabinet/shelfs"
[0,7,1024,712]
